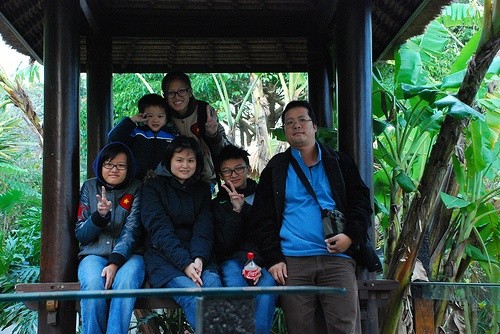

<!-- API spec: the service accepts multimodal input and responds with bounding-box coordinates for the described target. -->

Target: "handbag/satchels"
[322,210,344,245]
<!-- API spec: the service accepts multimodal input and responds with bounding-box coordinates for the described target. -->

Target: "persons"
[106,73,229,199]
[141,138,224,334]
[252,101,383,334]
[189,144,278,334]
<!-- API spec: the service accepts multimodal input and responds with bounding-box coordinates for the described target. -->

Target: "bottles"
[242,252,258,286]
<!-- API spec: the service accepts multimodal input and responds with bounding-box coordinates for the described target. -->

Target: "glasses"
[165,88,191,100]
[102,162,128,170]
[283,118,313,126]
[220,167,247,177]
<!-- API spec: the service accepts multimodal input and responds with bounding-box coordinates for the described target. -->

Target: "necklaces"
[76,143,146,334]
